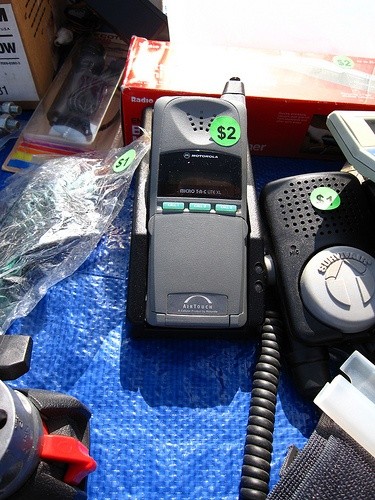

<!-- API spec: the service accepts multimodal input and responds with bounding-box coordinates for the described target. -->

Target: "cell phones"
[145,77,248,327]
